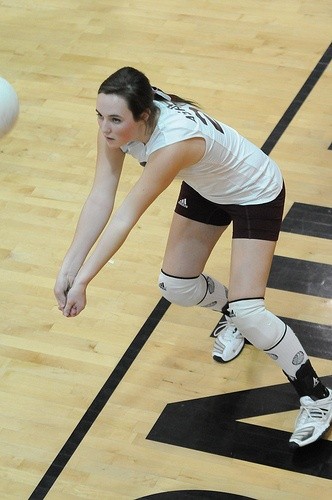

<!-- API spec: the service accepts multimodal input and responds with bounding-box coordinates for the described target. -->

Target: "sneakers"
[288,386,331,447]
[213,315,245,363]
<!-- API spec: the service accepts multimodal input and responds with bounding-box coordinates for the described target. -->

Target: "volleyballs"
[0,76,19,139]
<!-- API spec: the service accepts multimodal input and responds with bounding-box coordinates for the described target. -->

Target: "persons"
[54,67,332,450]
